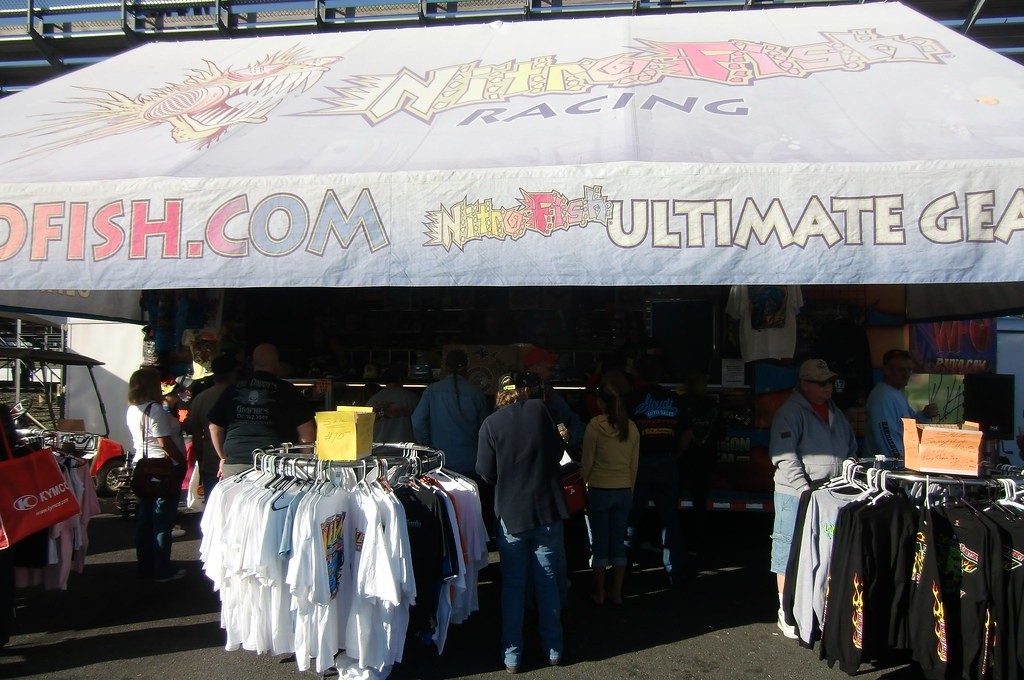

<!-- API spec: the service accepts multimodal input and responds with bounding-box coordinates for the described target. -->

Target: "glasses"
[807,380,832,387]
[537,363,556,372]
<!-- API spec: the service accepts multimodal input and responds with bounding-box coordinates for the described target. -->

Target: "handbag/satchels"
[130,458,178,499]
[0,420,80,551]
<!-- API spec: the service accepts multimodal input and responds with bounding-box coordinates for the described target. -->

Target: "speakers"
[963,374,1015,440]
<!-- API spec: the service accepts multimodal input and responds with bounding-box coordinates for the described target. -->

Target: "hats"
[799,359,839,381]
[524,347,561,370]
[498,371,524,393]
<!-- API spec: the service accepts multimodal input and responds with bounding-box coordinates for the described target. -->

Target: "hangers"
[819,453,1024,523]
[229,439,467,498]
[15,425,85,472]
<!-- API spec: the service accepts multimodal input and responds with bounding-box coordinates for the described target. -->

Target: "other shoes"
[154,569,186,582]
[777,608,799,640]
[171,525,186,537]
[548,660,559,665]
[506,667,518,674]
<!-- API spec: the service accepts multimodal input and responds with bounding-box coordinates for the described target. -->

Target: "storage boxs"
[903,417,982,478]
[315,404,376,462]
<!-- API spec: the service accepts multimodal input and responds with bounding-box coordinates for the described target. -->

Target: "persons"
[364,341,728,674]
[861,349,939,471]
[127,343,316,584]
[768,359,859,638]
